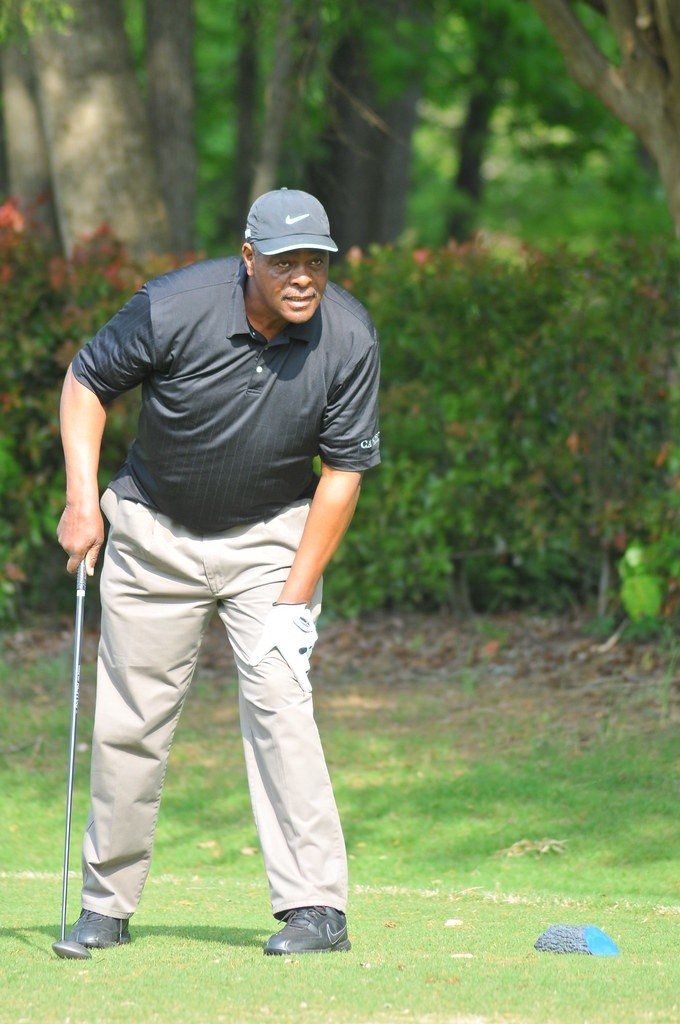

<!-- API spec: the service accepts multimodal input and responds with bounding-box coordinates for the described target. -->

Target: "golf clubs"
[51,556,95,962]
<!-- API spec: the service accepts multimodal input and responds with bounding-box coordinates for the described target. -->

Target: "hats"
[245,187,339,255]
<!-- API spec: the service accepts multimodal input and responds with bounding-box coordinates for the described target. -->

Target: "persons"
[55,187,383,956]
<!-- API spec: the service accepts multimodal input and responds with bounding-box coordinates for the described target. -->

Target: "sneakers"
[69,908,131,948]
[264,905,351,955]
